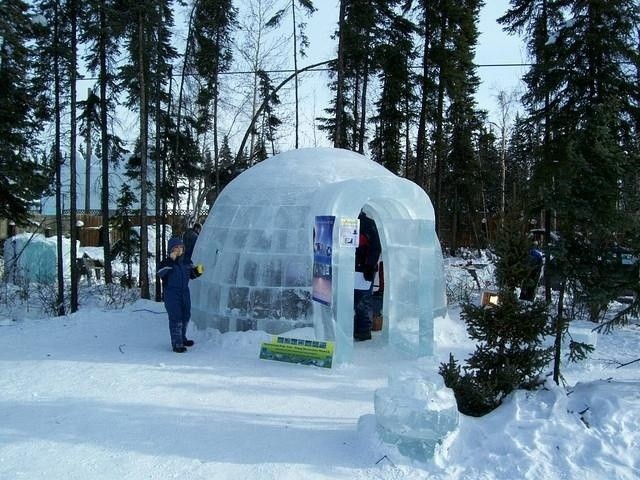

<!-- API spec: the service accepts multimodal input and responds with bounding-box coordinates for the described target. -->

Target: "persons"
[519,241,543,301]
[156,237,203,353]
[183,222,202,260]
[355,208,381,340]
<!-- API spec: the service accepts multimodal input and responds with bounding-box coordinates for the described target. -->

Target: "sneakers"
[172,340,194,352]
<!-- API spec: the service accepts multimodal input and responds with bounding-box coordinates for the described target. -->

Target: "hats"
[167,238,186,251]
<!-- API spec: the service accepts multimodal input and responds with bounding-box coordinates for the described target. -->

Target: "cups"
[197,264,203,273]
